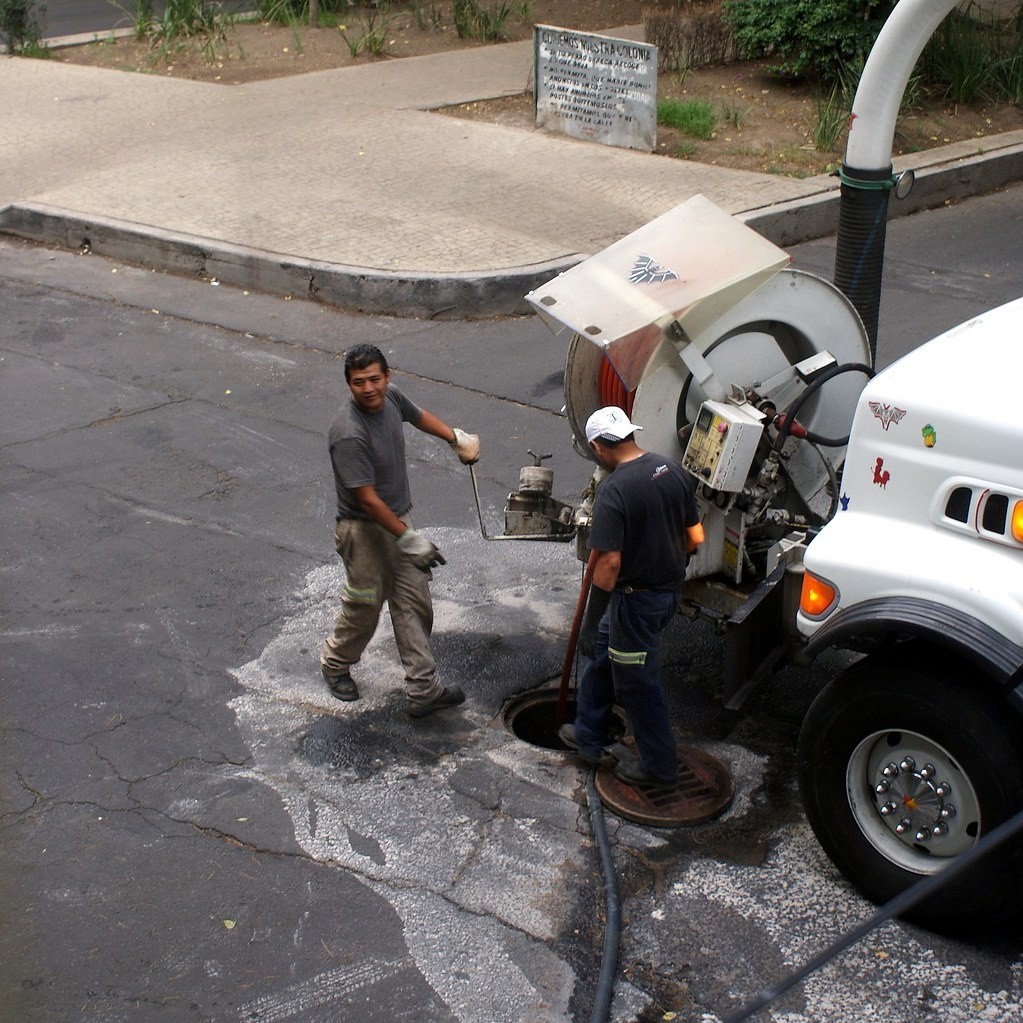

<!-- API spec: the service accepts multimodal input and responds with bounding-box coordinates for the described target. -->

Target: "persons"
[322,344,480,716]
[556,406,704,788]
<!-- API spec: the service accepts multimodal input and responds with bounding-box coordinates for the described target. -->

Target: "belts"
[616,586,654,593]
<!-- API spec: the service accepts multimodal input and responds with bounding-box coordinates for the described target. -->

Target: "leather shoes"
[323,668,356,700]
[614,760,679,790]
[407,685,466,719]
[559,723,600,762]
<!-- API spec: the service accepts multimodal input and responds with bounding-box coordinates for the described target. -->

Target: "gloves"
[395,530,446,574]
[449,428,480,465]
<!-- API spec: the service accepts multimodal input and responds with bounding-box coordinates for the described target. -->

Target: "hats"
[585,406,635,443]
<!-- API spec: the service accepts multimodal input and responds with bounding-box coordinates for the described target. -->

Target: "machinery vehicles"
[707,295,1023,945]
